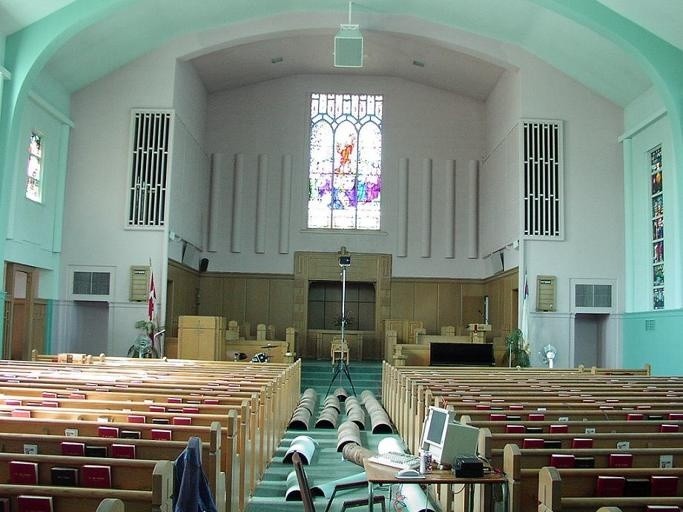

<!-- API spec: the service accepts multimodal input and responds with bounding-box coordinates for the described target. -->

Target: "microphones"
[478,309,488,324]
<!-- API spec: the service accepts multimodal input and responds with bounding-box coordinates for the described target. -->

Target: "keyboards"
[368,453,421,470]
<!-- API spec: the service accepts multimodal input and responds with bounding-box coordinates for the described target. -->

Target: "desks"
[363,457,510,512]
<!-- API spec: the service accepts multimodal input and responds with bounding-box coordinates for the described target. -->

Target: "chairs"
[291,451,385,512]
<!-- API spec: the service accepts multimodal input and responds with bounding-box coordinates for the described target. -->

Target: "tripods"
[324,267,359,399]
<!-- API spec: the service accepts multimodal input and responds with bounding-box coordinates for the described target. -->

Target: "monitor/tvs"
[423,406,479,467]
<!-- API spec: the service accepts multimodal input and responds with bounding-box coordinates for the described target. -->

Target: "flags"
[521,275,531,356]
[148,271,158,320]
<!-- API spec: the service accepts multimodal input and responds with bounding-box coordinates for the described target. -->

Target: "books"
[0,372,255,511]
[71,354,86,365]
[414,373,683,512]
[58,352,72,363]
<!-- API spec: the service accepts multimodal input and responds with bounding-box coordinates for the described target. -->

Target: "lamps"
[333,0,364,67]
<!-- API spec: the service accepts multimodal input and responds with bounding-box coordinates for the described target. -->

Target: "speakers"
[199,258,209,272]
[334,30,364,67]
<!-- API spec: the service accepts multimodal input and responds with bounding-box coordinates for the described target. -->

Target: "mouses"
[399,469,419,476]
